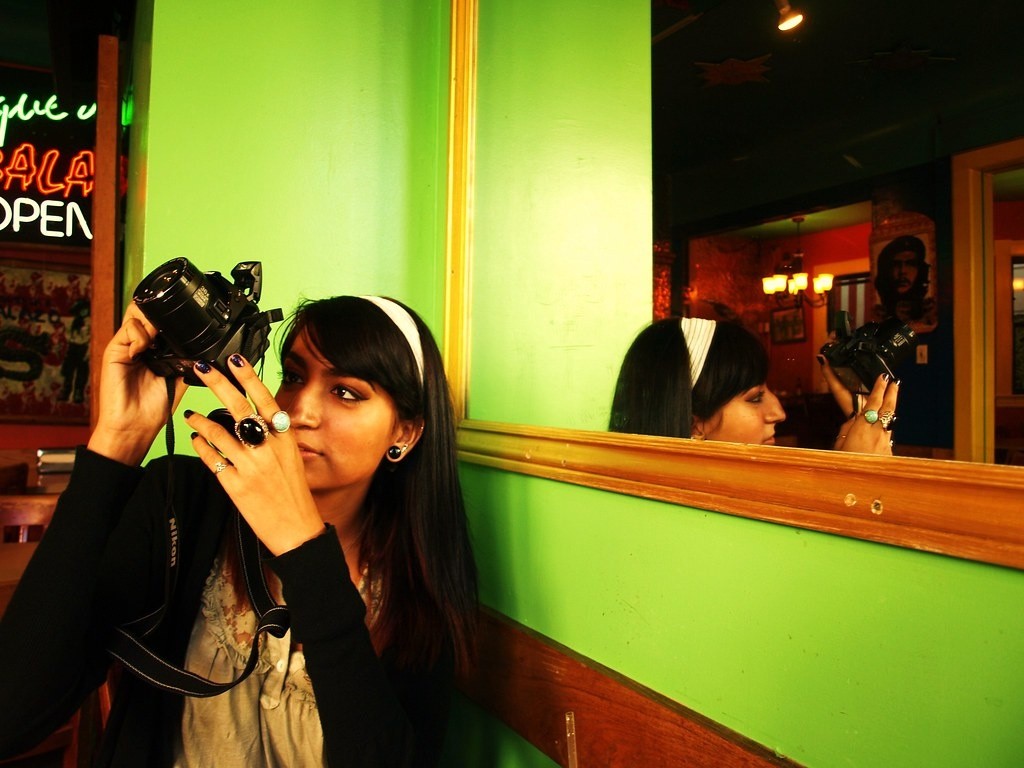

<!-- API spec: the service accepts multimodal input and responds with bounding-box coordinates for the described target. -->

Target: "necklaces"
[342,520,367,555]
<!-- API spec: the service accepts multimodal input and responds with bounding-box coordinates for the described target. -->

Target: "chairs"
[0,444,125,768]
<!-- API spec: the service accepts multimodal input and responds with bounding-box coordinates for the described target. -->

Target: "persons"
[0,296,481,768]
[609,317,902,457]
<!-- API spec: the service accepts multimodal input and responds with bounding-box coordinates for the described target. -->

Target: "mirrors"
[442,0,1024,573]
[826,271,884,345]
[950,136,1024,468]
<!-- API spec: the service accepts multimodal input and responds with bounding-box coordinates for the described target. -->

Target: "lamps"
[774,0,804,31]
[761,214,834,309]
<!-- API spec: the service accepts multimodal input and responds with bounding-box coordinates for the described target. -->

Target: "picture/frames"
[769,305,808,346]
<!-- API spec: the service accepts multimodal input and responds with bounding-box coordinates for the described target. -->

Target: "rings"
[879,410,897,432]
[214,461,229,475]
[889,440,893,446]
[272,411,291,433]
[837,435,846,439]
[865,411,879,423]
[235,414,270,448]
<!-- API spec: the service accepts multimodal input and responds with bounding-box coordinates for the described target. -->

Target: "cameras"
[818,317,918,395]
[131,257,284,387]
